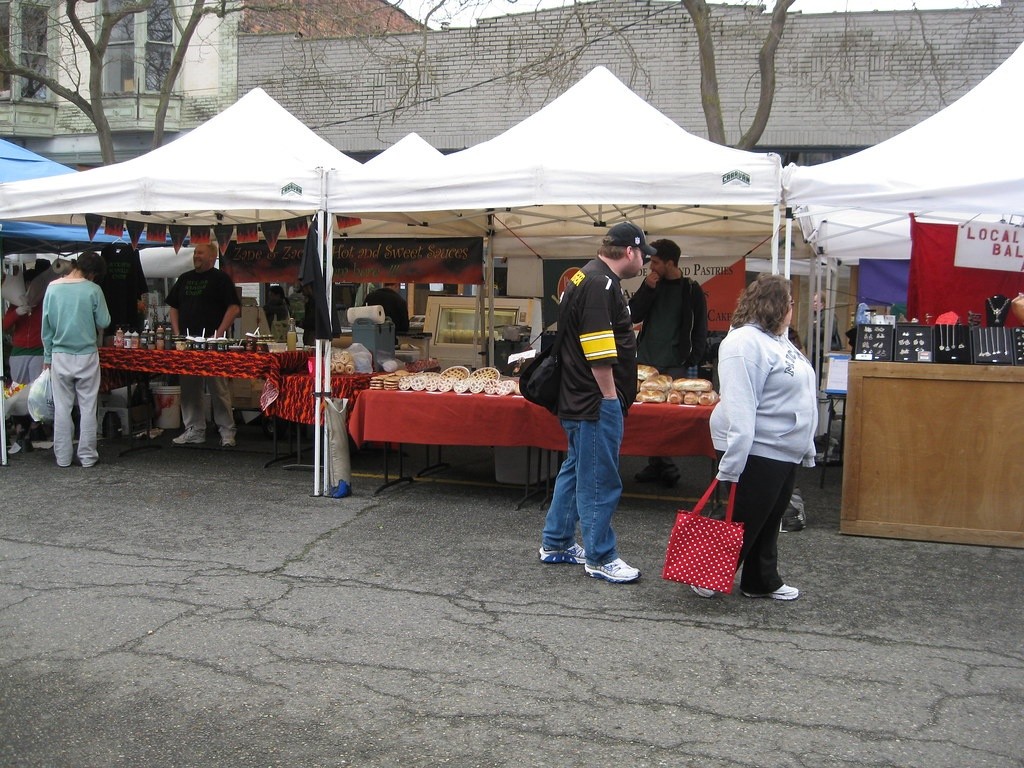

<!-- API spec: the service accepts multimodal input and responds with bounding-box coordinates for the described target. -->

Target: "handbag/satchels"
[830,329,841,351]
[345,343,373,375]
[663,477,745,594]
[518,343,560,414]
[28,367,55,421]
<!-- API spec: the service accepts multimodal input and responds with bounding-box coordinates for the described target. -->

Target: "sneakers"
[172,427,206,443]
[220,428,237,447]
[742,584,800,600]
[538,543,586,564]
[585,558,642,584]
[689,585,715,597]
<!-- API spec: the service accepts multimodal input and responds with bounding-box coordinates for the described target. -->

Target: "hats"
[605,222,657,256]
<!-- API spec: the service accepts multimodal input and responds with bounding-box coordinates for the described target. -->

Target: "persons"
[262,286,290,332]
[803,290,837,419]
[2,269,54,454]
[690,272,820,601]
[288,280,316,346]
[538,221,657,583]
[362,282,410,345]
[165,241,242,448]
[40,252,112,468]
[628,238,708,381]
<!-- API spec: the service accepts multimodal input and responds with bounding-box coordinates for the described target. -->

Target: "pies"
[370,369,409,390]
[398,369,518,396]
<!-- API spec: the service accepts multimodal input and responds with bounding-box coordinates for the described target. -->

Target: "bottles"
[131,331,139,348]
[287,325,296,351]
[123,330,131,348]
[140,325,274,352]
[306,357,324,376]
[115,327,123,348]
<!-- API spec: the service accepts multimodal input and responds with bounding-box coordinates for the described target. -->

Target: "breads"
[634,364,719,405]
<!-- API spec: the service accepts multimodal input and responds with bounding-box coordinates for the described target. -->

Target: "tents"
[0,40,1024,533]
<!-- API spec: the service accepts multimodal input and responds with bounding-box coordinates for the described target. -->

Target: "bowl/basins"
[267,343,287,353]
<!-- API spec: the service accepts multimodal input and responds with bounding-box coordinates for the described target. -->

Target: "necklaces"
[951,324,955,349]
[1003,326,1008,355]
[990,327,996,354]
[939,323,944,350]
[978,328,985,356]
[987,298,1010,323]
[996,327,1001,354]
[984,329,991,356]
[958,324,965,349]
[945,324,950,351]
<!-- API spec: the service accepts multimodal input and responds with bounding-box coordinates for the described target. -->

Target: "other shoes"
[8,441,53,454]
[635,464,681,485]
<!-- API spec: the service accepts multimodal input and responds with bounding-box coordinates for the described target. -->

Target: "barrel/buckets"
[152,386,182,429]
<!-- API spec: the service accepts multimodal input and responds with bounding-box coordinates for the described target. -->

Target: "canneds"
[185,332,269,353]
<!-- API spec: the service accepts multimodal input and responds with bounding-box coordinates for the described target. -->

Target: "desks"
[348,388,547,510]
[268,361,443,469]
[537,396,719,512]
[97,346,315,468]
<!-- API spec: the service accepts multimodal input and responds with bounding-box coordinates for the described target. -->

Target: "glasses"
[790,300,795,305]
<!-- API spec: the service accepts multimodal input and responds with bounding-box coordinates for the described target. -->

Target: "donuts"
[330,354,355,374]
